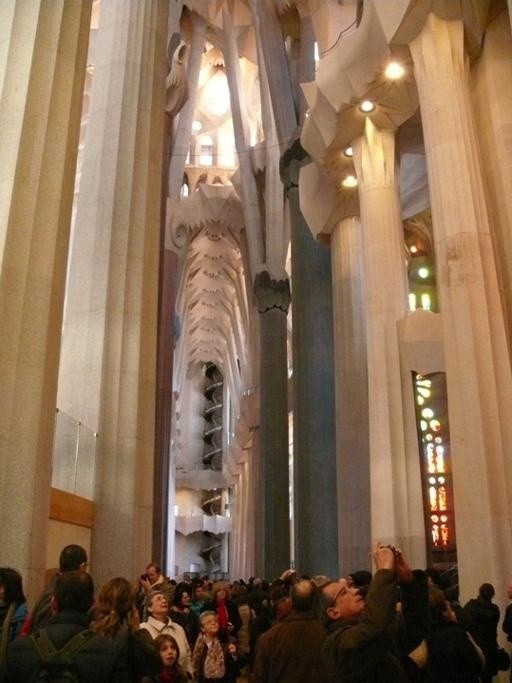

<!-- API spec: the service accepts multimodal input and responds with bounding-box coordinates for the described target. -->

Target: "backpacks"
[30,628,91,681]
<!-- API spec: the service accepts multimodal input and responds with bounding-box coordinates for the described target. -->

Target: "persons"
[2,541,512,682]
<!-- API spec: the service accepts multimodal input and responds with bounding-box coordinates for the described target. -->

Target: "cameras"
[141,574,150,581]
[383,544,399,561]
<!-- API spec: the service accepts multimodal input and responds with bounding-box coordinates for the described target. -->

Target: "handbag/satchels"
[497,646,509,671]
